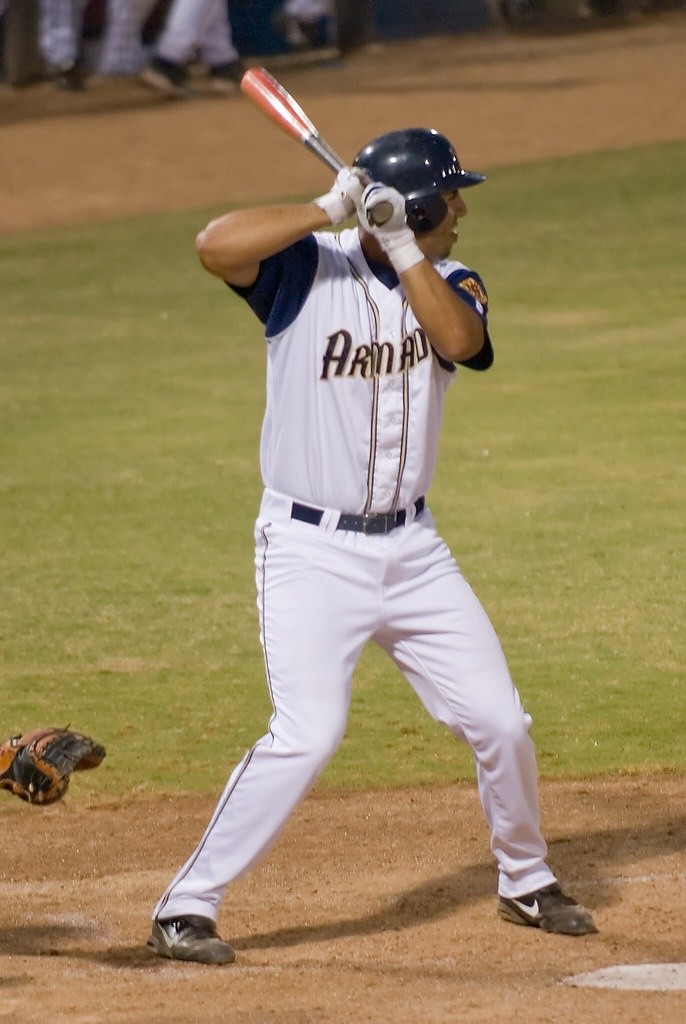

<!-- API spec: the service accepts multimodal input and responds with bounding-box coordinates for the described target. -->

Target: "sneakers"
[497,883,596,935]
[146,916,234,964]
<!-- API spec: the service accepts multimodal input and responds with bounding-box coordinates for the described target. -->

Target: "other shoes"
[140,60,195,98]
[47,63,76,89]
[86,74,121,89]
[212,64,245,94]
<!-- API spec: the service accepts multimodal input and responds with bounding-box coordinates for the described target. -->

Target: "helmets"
[352,127,485,201]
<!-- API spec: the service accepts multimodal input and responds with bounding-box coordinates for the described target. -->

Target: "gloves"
[357,183,425,273]
[312,165,369,226]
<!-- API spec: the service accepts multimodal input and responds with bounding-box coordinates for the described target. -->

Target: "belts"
[290,496,426,533]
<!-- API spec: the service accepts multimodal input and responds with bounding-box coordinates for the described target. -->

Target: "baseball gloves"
[0,727,105,805]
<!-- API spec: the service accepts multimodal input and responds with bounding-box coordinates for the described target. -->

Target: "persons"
[0,1,390,102]
[1,726,108,806]
[147,127,600,964]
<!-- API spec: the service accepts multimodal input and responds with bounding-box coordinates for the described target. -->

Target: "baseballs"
[240,65,394,226]
